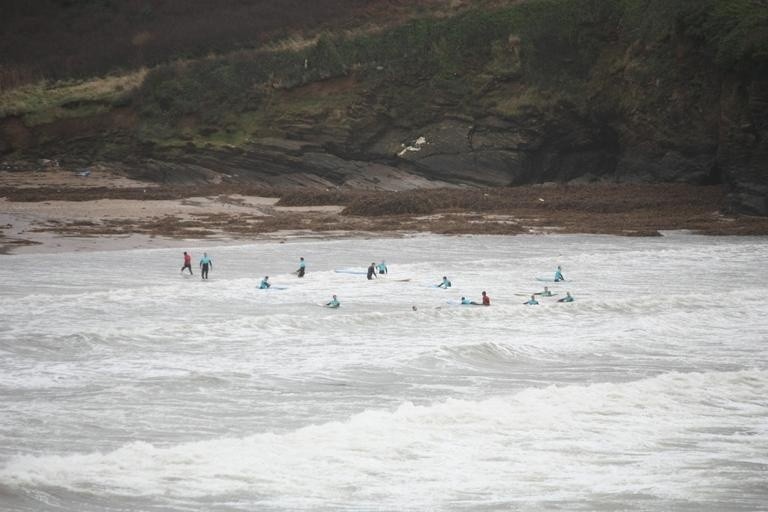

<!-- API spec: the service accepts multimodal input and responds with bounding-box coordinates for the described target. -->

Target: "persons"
[439,276,450,287]
[476,291,491,305]
[532,286,557,297]
[461,296,476,304]
[554,265,564,282]
[180,252,192,275]
[412,305,418,311]
[375,260,387,274]
[198,253,212,279]
[261,276,267,290]
[366,262,375,280]
[326,296,339,308]
[296,257,306,277]
[522,295,538,305]
[557,291,575,302]
